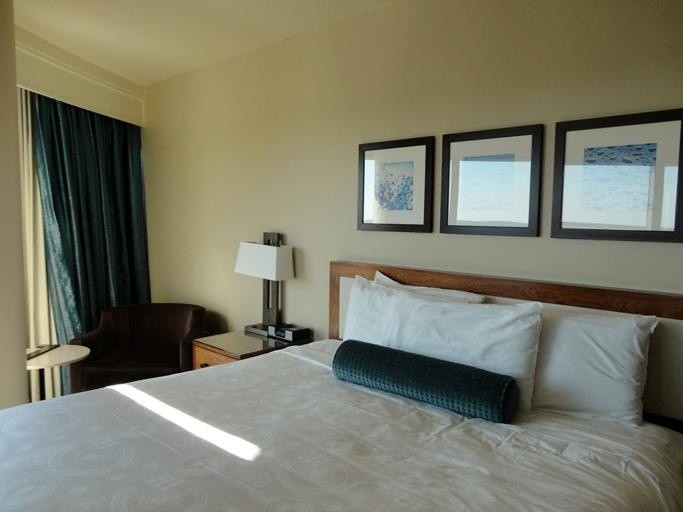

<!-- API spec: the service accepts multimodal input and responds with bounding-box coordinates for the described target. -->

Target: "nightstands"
[191,330,266,371]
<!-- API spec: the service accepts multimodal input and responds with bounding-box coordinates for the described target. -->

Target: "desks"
[25,343,90,401]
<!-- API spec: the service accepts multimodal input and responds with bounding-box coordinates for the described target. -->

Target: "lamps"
[233,231,296,337]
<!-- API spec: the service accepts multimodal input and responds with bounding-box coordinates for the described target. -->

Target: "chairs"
[68,303,208,394]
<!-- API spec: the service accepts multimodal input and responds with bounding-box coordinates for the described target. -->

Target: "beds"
[0,260,682,512]
